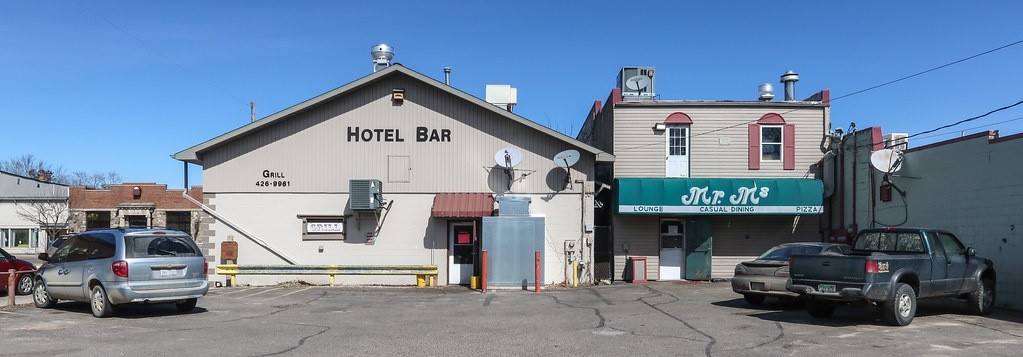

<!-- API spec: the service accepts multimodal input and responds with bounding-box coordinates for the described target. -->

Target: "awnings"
[614,178,824,216]
[431,191,496,218]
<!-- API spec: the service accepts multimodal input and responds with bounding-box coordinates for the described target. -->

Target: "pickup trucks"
[785,226,997,326]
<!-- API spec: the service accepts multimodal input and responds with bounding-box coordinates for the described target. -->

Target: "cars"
[0,248,38,296]
[42,234,101,266]
[731,242,851,309]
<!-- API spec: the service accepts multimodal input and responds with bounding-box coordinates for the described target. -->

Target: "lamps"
[393,88,404,100]
[655,123,667,131]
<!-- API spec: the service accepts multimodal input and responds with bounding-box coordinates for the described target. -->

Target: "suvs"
[32,226,210,319]
